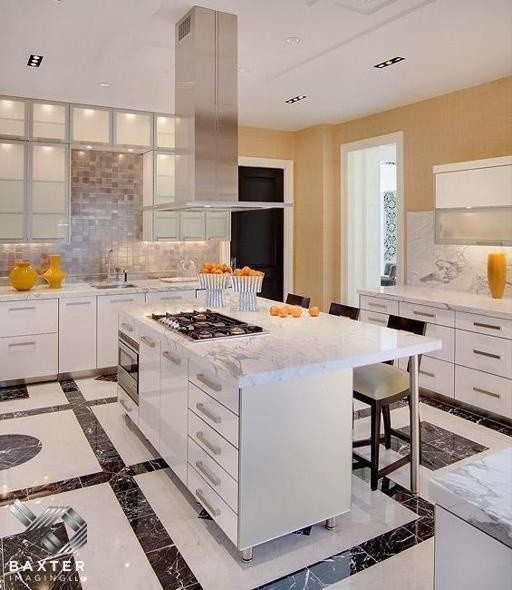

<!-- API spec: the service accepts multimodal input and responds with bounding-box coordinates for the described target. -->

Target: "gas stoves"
[145,308,271,341]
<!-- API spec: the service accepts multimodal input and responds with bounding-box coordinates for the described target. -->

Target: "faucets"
[105,249,114,282]
[177,260,186,276]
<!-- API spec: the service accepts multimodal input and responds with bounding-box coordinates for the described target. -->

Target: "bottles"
[8,255,66,291]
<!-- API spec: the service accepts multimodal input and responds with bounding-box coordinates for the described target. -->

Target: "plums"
[199,262,261,276]
[269,305,319,317]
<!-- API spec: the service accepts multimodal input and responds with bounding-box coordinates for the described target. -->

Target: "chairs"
[353,315,427,492]
[286,293,360,320]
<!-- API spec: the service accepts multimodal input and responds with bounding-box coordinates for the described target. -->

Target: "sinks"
[160,277,199,283]
[94,282,137,290]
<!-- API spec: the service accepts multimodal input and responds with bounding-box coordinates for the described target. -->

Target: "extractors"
[138,6,293,214]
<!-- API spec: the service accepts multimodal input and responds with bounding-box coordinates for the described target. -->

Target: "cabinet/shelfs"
[0,299,59,388]
[71,103,153,154]
[117,312,354,563]
[58,287,242,382]
[0,97,70,244]
[433,155,512,248]
[359,295,512,428]
[142,112,232,241]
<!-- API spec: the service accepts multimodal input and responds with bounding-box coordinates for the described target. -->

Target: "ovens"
[116,328,139,407]
